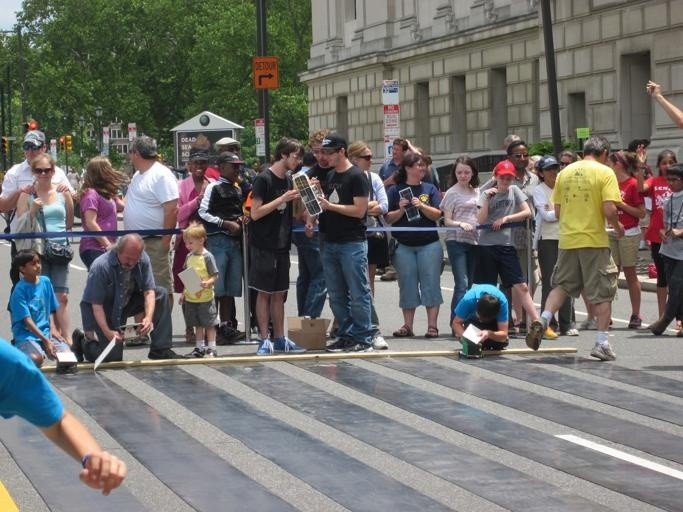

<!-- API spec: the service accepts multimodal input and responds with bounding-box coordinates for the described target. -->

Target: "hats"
[628,139,650,151]
[216,137,240,146]
[189,147,209,161]
[320,133,347,150]
[216,151,245,165]
[404,154,432,167]
[539,155,560,169]
[495,160,516,177]
[134,136,158,157]
[23,130,45,146]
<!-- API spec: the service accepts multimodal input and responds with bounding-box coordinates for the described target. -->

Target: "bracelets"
[81,452,91,469]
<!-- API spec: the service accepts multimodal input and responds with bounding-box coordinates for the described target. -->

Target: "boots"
[186,328,196,343]
[649,313,672,335]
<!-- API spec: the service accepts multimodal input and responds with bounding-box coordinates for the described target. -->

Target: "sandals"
[425,327,438,338]
[393,325,414,337]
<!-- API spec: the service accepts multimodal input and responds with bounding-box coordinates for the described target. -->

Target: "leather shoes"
[71,328,85,363]
[149,348,183,360]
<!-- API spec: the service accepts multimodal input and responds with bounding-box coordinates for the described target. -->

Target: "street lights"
[77,115,86,170]
[94,104,103,155]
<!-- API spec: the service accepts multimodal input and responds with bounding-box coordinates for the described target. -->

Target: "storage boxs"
[287,315,332,351]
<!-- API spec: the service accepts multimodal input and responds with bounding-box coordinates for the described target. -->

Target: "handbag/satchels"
[44,240,73,263]
[377,237,389,269]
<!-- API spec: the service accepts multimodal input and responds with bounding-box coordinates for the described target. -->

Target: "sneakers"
[326,337,389,352]
[566,328,579,335]
[274,336,307,353]
[125,335,149,346]
[203,349,217,358]
[184,348,205,358]
[543,327,558,340]
[380,270,397,281]
[628,315,641,328]
[590,343,617,361]
[257,336,274,356]
[526,317,547,350]
[205,324,246,345]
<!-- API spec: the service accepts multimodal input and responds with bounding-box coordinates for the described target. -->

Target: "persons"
[377,135,682,361]
[2,130,388,367]
[644,80,681,130]
[0,333,127,496]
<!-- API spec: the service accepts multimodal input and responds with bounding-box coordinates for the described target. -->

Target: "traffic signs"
[384,127,398,159]
[381,79,398,87]
[252,56,279,89]
[383,104,400,128]
[382,87,399,103]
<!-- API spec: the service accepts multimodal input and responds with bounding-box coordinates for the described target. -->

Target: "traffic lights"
[0,137,7,154]
[29,118,37,129]
[59,135,65,151]
[65,134,73,151]
[20,122,29,139]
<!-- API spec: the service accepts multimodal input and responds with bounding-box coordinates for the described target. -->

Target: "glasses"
[34,168,52,173]
[507,154,528,158]
[119,323,142,333]
[23,144,41,150]
[560,162,569,167]
[359,155,371,161]
[228,147,240,152]
